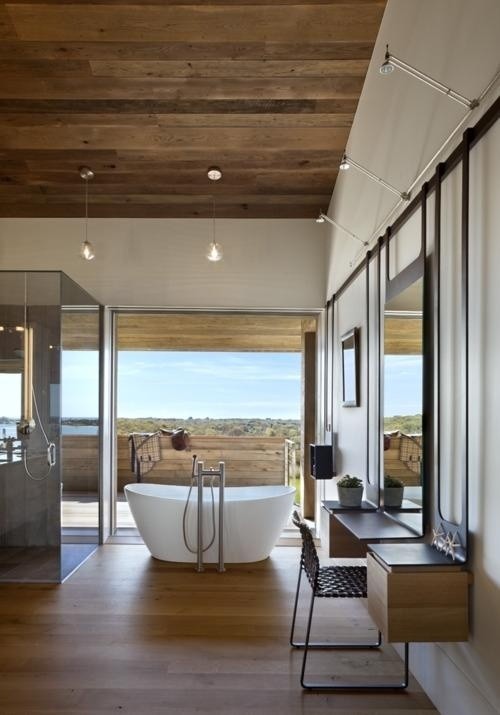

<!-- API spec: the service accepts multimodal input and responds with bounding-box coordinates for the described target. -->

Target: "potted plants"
[384,473,404,508]
[337,474,364,507]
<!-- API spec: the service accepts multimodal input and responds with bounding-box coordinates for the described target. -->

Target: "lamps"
[316,209,367,247]
[379,44,471,110]
[77,166,96,259]
[340,154,406,200]
[206,166,224,262]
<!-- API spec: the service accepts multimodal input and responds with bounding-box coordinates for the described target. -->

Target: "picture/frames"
[340,327,358,408]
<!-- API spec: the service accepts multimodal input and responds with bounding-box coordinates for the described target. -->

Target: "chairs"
[289,511,408,689]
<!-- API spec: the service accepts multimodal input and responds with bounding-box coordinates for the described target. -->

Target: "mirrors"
[377,251,426,536]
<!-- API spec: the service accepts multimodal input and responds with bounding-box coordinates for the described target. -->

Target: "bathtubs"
[122,481,296,563]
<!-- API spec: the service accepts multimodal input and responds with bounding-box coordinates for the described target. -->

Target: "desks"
[320,500,469,643]
